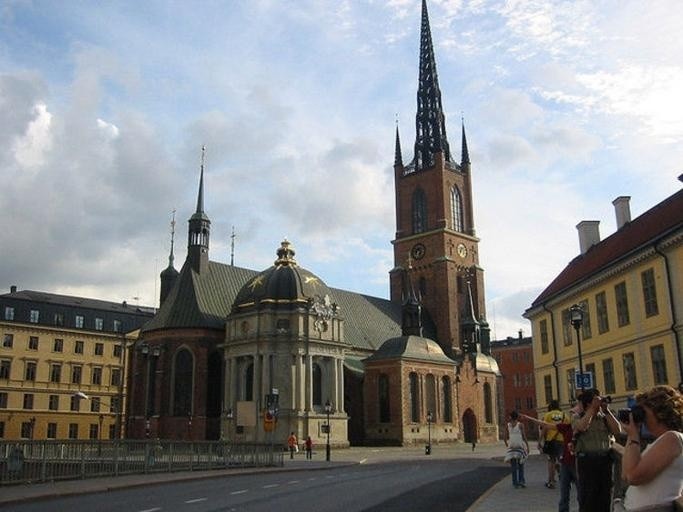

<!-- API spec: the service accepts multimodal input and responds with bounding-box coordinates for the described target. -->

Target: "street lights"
[226,409,234,441]
[324,399,332,461]
[570,304,587,394]
[98,415,104,456]
[141,343,160,438]
[426,411,433,455]
[75,392,120,439]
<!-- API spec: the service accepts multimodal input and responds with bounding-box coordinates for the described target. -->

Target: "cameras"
[618,405,646,424]
[601,396,612,403]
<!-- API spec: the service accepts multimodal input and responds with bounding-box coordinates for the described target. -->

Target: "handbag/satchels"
[543,440,556,454]
[613,498,626,512]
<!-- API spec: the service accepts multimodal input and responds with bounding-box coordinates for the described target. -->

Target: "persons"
[612,384,683,512]
[518,389,583,512]
[305,437,312,460]
[287,432,297,459]
[503,411,530,487]
[569,386,622,512]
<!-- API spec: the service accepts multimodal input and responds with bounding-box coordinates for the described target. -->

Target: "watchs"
[624,439,641,447]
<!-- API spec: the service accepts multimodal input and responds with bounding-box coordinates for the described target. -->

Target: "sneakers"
[545,481,554,488]
[514,483,527,489]
